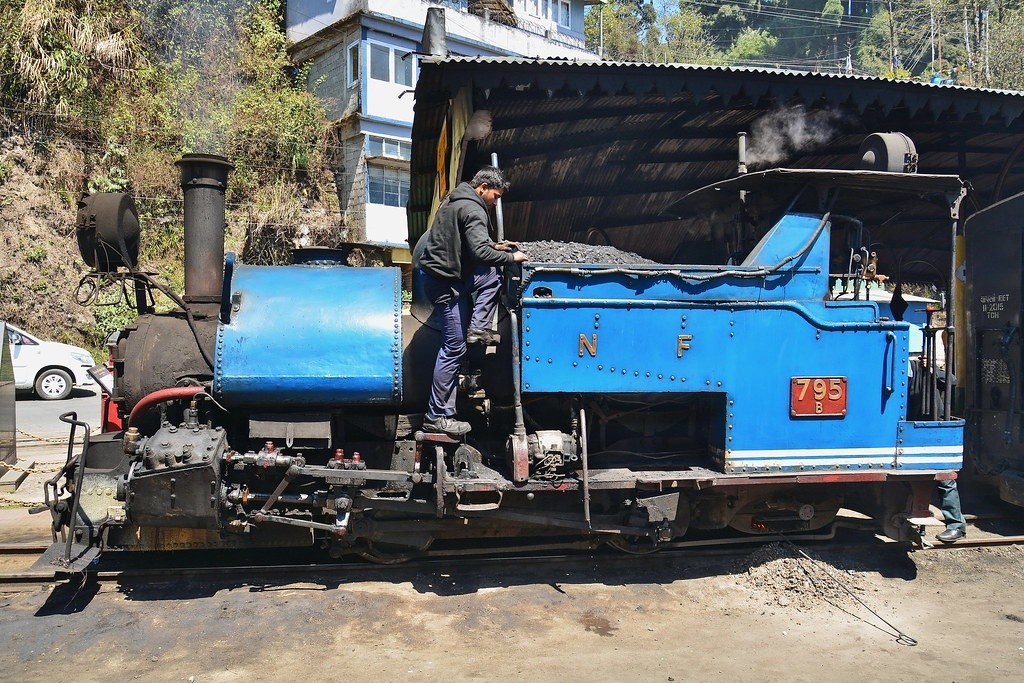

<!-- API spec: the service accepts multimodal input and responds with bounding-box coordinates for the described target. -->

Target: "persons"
[415,164,529,437]
[908,359,966,542]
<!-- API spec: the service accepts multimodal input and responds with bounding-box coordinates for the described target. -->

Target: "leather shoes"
[423,413,472,436]
[467,329,500,346]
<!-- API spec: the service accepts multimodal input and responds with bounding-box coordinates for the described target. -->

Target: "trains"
[28,151,966,577]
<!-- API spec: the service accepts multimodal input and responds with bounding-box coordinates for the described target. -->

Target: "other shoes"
[935,530,967,542]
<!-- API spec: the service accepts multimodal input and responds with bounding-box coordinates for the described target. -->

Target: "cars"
[6,322,96,400]
